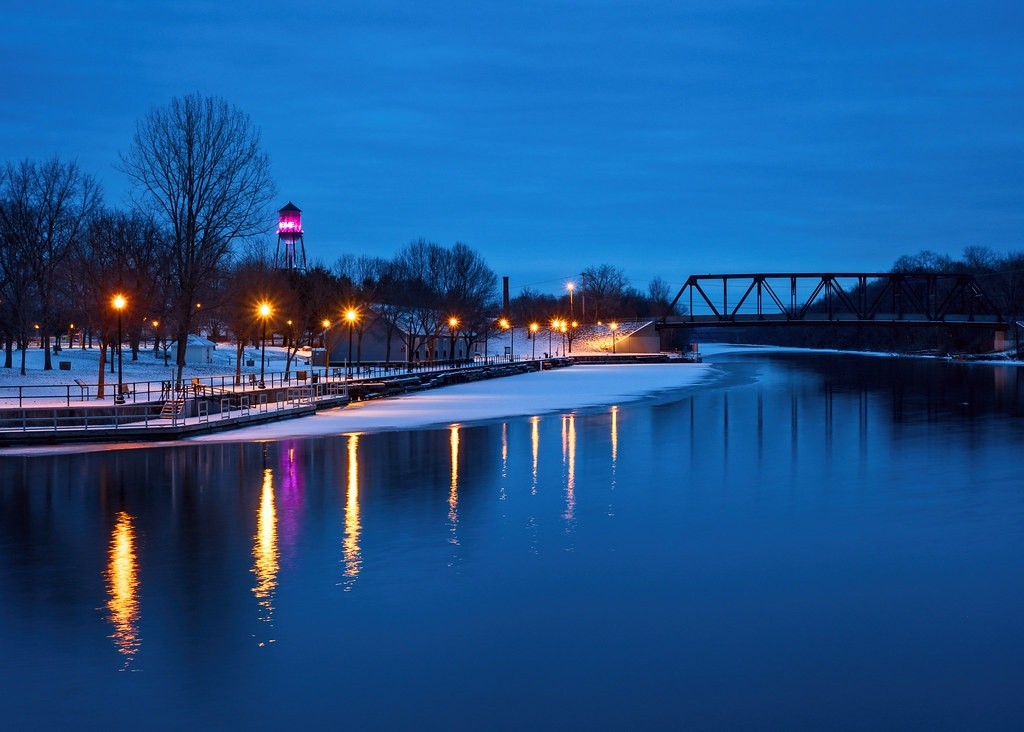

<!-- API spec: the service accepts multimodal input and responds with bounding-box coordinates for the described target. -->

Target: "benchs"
[118,367,406,398]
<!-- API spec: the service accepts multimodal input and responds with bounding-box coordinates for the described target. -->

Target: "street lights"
[342,305,360,379]
[447,317,460,368]
[256,300,274,388]
[499,314,617,361]
[110,294,128,403]
